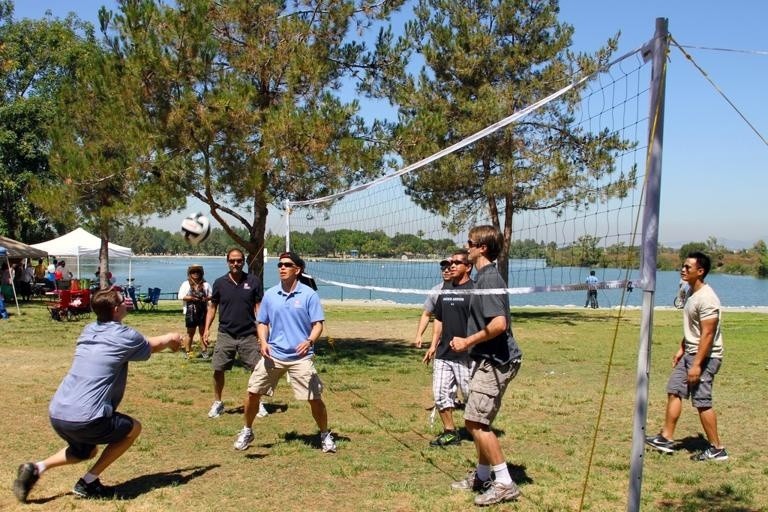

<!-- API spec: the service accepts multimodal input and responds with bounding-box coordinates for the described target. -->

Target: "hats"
[280,252,305,272]
[188,264,203,272]
[440,257,452,265]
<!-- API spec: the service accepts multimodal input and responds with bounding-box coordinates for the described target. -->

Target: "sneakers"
[73,477,114,499]
[320,431,336,453]
[184,351,189,359]
[430,429,462,445]
[690,445,727,461]
[474,481,521,505]
[208,400,225,418]
[255,401,268,418]
[233,425,255,451]
[644,434,675,453]
[13,462,39,502]
[200,350,209,359]
[451,470,494,492]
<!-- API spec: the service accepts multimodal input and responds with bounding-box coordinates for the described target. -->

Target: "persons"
[678,277,688,306]
[233,251,337,452]
[627,280,633,292]
[414,225,523,507]
[13,286,179,505]
[645,253,728,461]
[181,265,212,359]
[0,256,73,303]
[203,248,268,418]
[584,271,598,307]
[298,262,317,292]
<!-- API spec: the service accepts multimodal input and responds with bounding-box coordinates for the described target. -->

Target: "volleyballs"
[181,213,211,244]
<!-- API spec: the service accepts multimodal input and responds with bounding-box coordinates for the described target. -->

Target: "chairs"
[126,285,161,311]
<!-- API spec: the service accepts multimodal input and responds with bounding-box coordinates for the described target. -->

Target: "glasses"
[228,259,243,263]
[278,262,293,267]
[441,265,450,270]
[468,240,479,248]
[450,260,466,265]
[681,263,690,270]
